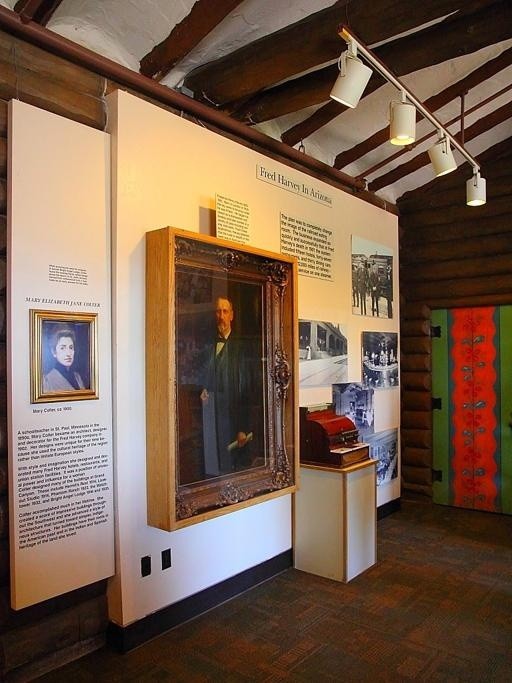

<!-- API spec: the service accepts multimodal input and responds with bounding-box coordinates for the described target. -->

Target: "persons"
[355,261,367,315]
[368,264,380,317]
[385,263,392,318]
[42,328,85,391]
[195,289,259,479]
[363,349,399,388]
[351,262,359,306]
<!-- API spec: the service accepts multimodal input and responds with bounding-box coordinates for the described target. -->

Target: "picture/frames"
[28,309,99,404]
[145,226,302,533]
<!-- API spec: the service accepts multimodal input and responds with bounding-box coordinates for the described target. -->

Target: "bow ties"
[216,336,226,344]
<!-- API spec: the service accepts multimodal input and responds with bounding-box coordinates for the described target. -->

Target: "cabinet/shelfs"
[293,459,380,584]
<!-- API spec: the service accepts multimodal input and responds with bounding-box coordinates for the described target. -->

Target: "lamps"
[388,89,416,146]
[465,164,487,207]
[427,128,458,178]
[329,41,373,109]
